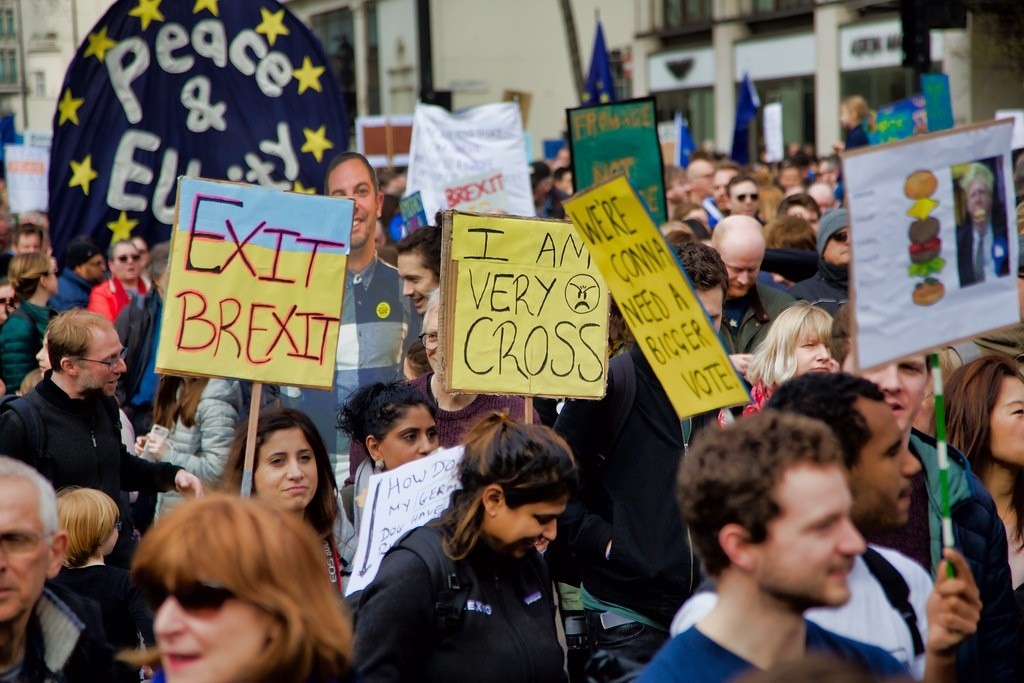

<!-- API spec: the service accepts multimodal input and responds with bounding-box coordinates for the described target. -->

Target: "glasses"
[112,522,123,531]
[112,254,142,263]
[44,267,59,276]
[731,194,760,202]
[0,531,56,552]
[142,574,238,618]
[74,346,128,372]
[829,231,848,243]
[418,331,439,350]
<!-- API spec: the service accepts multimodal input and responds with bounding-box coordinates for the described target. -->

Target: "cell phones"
[140,424,169,462]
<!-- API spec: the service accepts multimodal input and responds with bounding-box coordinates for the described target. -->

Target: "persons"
[957,163,1009,288]
[0,94,1024,682]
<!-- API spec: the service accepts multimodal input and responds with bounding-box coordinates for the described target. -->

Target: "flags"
[673,112,694,169]
[730,74,761,166]
[580,21,619,107]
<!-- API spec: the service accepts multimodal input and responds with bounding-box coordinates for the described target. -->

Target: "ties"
[975,233,984,283]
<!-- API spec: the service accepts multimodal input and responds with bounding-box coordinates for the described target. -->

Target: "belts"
[564,620,585,636]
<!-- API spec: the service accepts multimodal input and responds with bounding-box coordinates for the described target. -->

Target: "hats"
[817,208,849,258]
[61,241,98,265]
